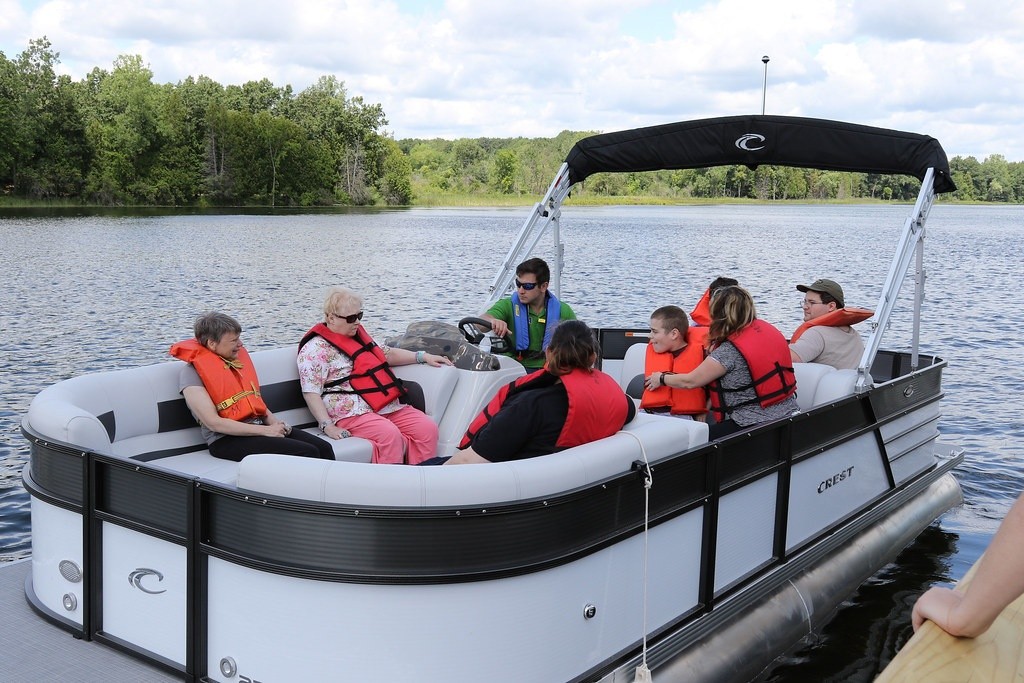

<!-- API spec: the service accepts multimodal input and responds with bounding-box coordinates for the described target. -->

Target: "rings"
[339,435,341,438]
[444,356,448,359]
[341,431,348,438]
[347,431,349,432]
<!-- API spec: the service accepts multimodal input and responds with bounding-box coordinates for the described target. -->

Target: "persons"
[179,311,336,462]
[691,277,738,327]
[644,287,800,442]
[789,279,865,370]
[416,319,636,467]
[297,291,454,464]
[476,258,577,373]
[912,490,1024,640]
[643,306,718,426]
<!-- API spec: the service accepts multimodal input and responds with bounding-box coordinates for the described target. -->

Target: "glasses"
[800,300,824,308]
[332,311,363,324]
[516,279,537,291]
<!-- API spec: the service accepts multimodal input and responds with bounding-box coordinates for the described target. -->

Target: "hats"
[796,279,844,307]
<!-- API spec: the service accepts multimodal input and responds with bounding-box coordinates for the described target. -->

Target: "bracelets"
[321,420,332,432]
[415,351,425,364]
[660,373,670,386]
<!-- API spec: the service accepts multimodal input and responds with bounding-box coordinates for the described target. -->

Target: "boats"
[0,116,966,682]
[872,550,1024,683]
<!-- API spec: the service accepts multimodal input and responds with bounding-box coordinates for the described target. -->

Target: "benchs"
[241,411,710,508]
[30,344,461,485]
[618,341,858,410]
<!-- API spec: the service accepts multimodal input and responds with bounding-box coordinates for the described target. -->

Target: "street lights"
[758,55,769,115]
